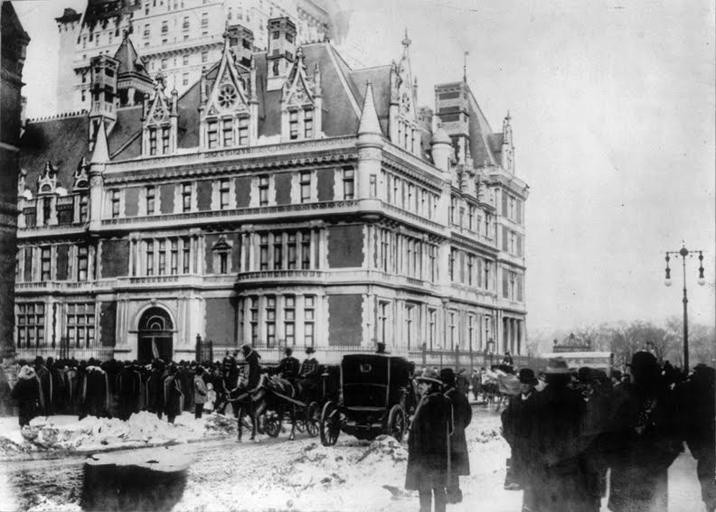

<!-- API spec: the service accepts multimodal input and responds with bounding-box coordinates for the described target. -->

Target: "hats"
[627,352,657,367]
[517,370,538,385]
[437,369,458,379]
[306,347,316,353]
[544,356,576,373]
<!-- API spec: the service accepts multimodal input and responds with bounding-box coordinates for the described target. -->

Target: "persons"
[7,346,318,430]
[406,367,472,511]
[500,351,715,511]
[471,351,514,401]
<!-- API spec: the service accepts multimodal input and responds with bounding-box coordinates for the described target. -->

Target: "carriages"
[320,351,470,447]
[480,364,520,410]
[223,343,341,438]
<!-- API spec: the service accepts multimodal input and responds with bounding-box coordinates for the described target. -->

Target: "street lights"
[665,240,706,374]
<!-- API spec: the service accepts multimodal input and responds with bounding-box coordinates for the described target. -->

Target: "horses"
[220,344,302,442]
